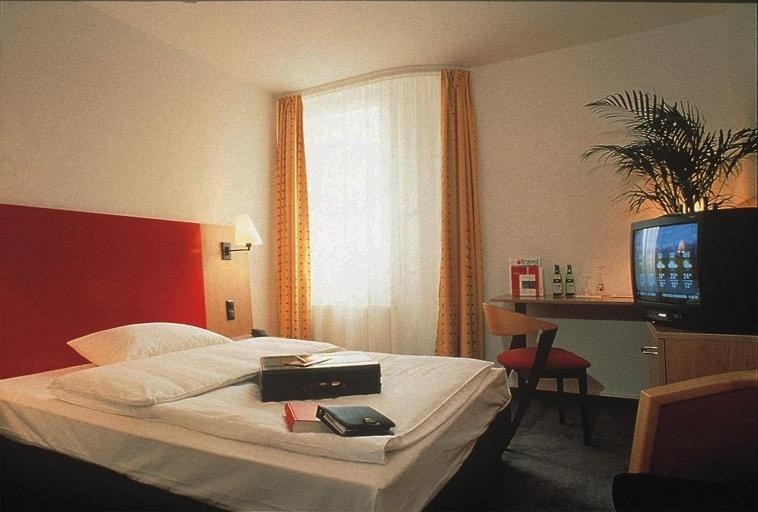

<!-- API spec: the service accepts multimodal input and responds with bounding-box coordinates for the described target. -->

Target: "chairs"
[481,303,592,450]
[612,370,758,512]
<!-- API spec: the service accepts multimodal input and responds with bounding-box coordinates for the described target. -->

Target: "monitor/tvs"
[628,205,756,333]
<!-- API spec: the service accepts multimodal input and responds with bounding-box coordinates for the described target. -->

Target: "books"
[315,405,395,437]
[284,402,333,432]
[509,255,545,297]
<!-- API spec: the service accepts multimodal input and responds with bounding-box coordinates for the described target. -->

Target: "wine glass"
[591,266,606,296]
[580,274,592,296]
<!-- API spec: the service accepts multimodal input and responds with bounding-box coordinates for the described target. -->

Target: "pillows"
[67,322,234,365]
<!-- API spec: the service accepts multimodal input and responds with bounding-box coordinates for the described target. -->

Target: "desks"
[489,292,758,409]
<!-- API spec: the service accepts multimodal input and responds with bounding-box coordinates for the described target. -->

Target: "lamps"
[221,214,263,260]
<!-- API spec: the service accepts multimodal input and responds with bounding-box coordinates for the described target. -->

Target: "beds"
[0,203,512,512]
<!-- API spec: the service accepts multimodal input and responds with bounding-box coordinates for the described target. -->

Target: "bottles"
[564,264,575,297]
[550,264,563,298]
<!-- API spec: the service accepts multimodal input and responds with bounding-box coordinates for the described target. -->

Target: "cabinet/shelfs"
[641,321,758,388]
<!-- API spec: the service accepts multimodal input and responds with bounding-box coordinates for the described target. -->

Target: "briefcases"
[260,353,382,402]
